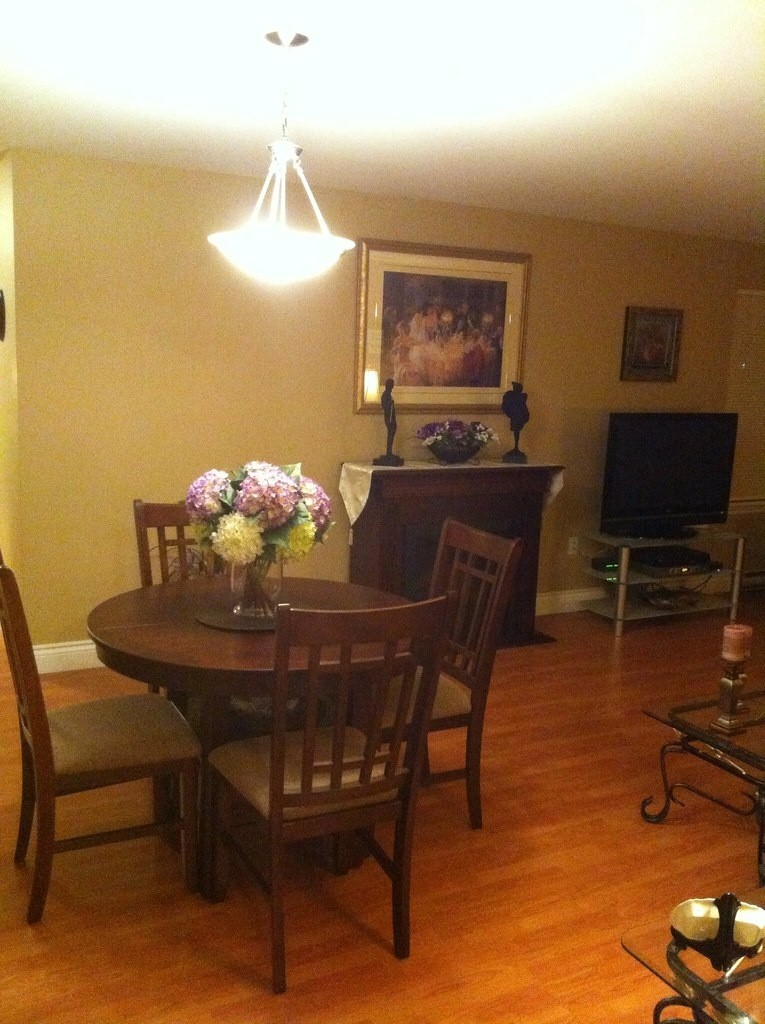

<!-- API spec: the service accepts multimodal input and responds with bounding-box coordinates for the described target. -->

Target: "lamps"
[202,31,357,296]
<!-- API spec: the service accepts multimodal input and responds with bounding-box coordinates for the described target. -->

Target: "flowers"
[414,417,502,449]
[184,460,332,567]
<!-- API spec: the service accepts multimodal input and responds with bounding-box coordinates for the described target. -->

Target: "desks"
[338,457,565,649]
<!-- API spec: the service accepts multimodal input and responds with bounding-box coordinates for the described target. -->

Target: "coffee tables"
[638,678,765,891]
[619,899,765,1024]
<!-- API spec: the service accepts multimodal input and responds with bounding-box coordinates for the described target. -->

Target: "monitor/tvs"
[599,413,738,533]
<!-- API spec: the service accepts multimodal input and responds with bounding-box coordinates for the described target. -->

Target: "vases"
[231,553,279,620]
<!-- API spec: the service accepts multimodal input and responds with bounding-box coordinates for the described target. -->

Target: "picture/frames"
[618,304,683,383]
[352,235,531,417]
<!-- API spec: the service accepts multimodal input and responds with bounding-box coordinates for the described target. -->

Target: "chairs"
[349,517,526,830]
[202,588,460,994]
[130,498,238,746]
[0,548,205,925]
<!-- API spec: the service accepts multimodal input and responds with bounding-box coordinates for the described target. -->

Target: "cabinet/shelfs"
[578,525,745,637]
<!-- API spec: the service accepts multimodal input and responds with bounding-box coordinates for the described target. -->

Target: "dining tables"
[85,575,420,898]
